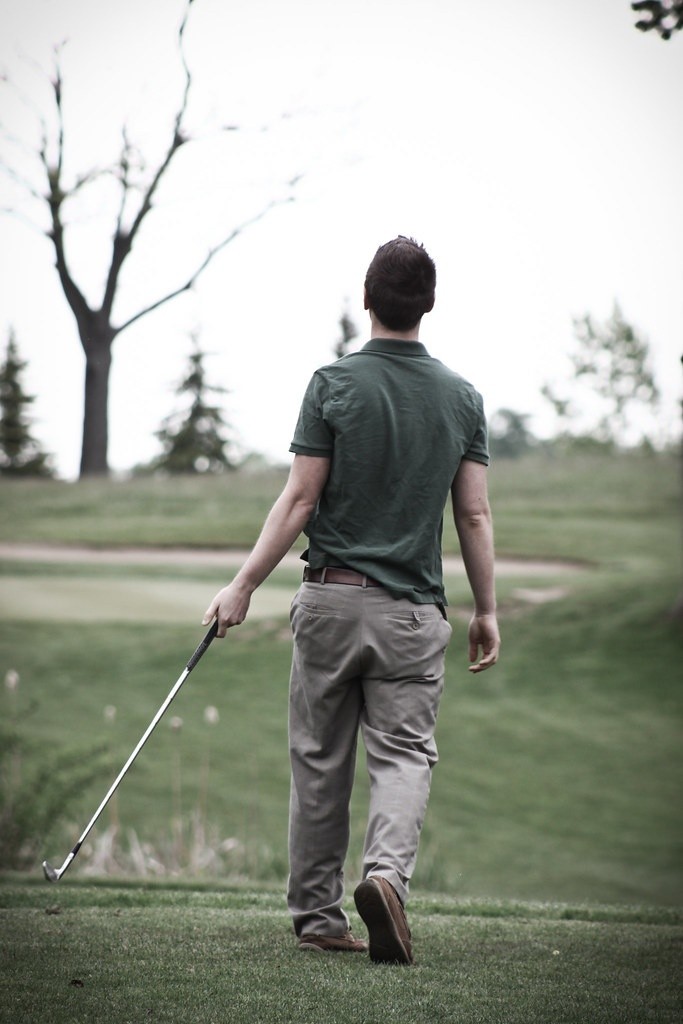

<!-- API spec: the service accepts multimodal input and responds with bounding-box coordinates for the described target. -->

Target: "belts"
[301,564,382,589]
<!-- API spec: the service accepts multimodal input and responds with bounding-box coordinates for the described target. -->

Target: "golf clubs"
[41,622,218,880]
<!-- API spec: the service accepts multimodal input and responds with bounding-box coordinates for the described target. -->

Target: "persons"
[201,235,500,965]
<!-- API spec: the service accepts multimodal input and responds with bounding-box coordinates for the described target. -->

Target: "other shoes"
[353,875,415,966]
[298,931,369,954]
